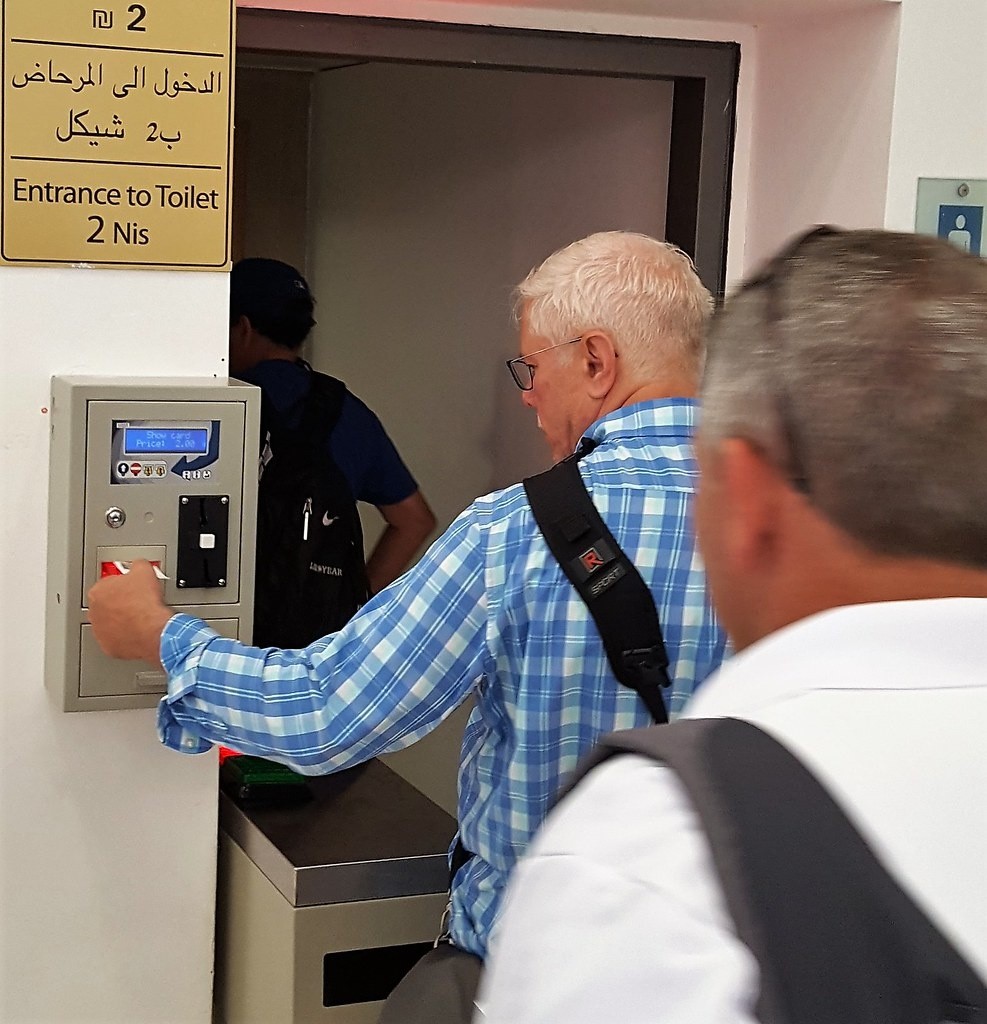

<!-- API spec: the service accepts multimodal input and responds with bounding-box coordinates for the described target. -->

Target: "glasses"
[506,336,619,392]
[736,222,847,493]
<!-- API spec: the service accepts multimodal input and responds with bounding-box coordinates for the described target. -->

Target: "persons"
[466,226,986,1024]
[86,229,734,1023]
[229,258,435,648]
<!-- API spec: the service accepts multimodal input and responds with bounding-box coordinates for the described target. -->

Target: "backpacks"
[251,370,377,648]
